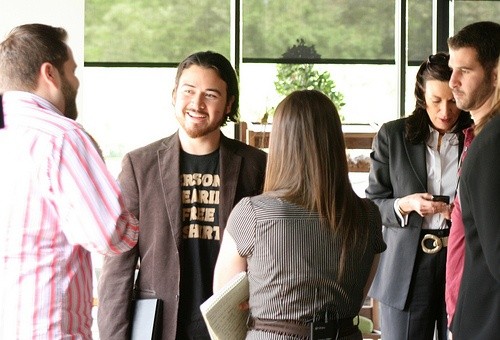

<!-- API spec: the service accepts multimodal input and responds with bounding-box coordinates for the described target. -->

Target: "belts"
[417,234,450,254]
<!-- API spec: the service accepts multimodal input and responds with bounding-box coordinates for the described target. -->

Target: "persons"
[446,22,500,340]
[0,24,140,340]
[365,52,474,340]
[97,50,268,340]
[212,90,387,340]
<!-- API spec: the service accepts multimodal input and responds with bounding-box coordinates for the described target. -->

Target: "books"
[200,271,249,340]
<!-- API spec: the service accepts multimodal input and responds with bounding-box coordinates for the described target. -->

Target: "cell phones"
[431,195,449,205]
